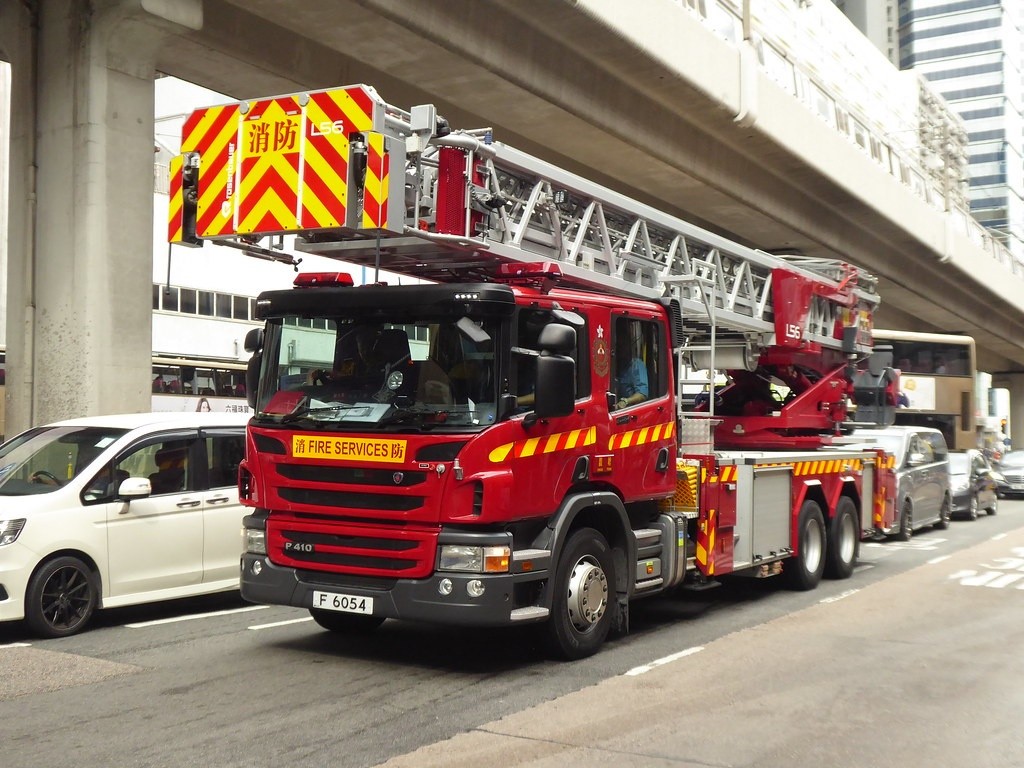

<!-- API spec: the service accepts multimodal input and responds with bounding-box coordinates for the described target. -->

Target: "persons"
[27,450,92,485]
[613,332,649,411]
[313,330,374,390]
[448,340,535,403]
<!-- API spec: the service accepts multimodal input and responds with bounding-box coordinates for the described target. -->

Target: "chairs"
[373,329,417,407]
[145,449,181,496]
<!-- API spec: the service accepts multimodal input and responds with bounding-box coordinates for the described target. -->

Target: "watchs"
[620,398,628,404]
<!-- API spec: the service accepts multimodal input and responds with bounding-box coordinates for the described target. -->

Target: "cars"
[992,447,1023,501]
[942,447,1000,520]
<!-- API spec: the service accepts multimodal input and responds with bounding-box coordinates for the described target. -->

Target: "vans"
[0,411,260,638]
[832,424,953,542]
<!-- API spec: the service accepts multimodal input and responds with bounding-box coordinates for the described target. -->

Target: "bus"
[868,329,987,465]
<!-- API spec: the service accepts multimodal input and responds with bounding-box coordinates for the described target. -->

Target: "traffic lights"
[1001,417,1010,437]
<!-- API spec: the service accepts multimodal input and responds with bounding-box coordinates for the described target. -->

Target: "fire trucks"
[163,83,914,664]
